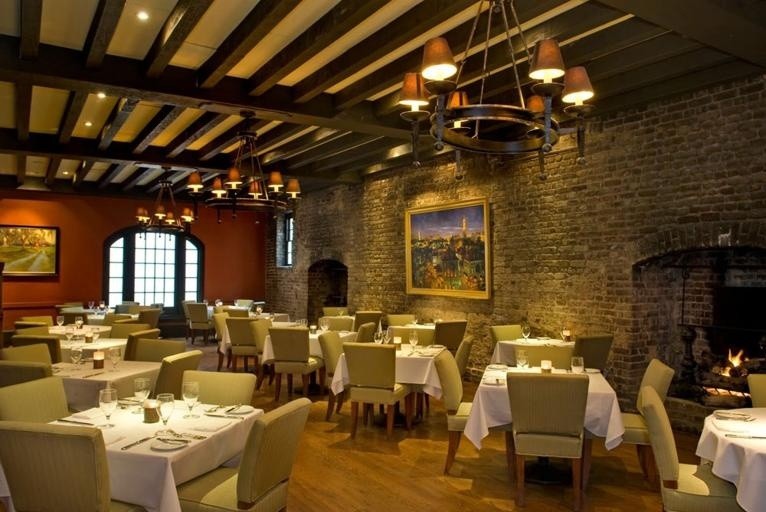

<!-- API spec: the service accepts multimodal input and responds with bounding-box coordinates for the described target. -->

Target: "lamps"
[399,0,598,183]
[135,111,301,240]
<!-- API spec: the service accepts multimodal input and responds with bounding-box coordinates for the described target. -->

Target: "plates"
[150,439,187,453]
[712,408,755,423]
[419,345,443,357]
[482,377,505,386]
[222,403,254,416]
[487,363,508,370]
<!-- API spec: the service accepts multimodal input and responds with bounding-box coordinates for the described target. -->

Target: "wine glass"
[521,323,532,345]
[515,350,529,371]
[559,325,568,344]
[201,296,419,353]
[96,377,203,434]
[55,301,110,370]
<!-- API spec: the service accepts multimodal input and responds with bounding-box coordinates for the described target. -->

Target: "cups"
[570,356,584,376]
[540,360,552,374]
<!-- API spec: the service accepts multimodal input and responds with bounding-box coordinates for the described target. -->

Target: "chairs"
[0,357,53,386]
[490,325,525,343]
[583,358,677,484]
[170,396,313,507]
[2,419,131,510]
[747,372,766,405]
[149,348,203,399]
[181,295,474,441]
[3,295,181,361]
[641,384,741,511]
[434,346,515,485]
[1,375,74,506]
[507,371,591,511]
[0,342,51,366]
[572,334,614,370]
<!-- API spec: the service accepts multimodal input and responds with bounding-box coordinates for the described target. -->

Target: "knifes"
[723,433,766,440]
[121,436,152,450]
[225,403,242,411]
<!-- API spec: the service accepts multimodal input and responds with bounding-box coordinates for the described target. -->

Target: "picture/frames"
[0,224,60,277]
[405,195,492,302]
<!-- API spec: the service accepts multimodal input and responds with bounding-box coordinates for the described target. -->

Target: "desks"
[40,393,266,510]
[47,357,163,409]
